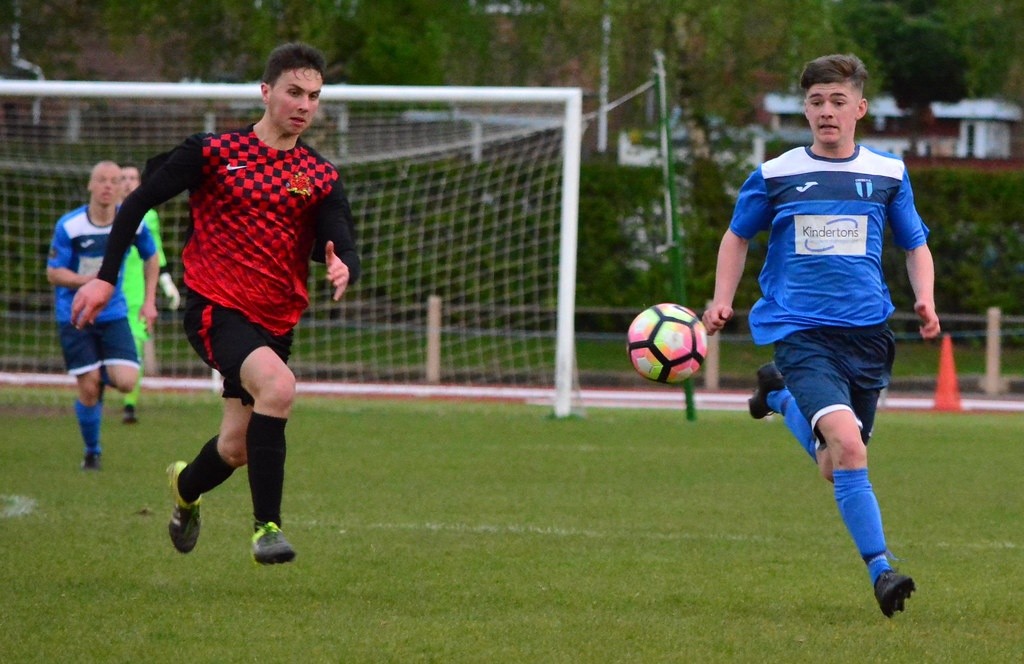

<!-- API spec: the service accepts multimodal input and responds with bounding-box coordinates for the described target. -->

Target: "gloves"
[160,272,181,311]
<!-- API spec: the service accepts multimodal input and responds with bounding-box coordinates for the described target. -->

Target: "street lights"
[933,332,963,413]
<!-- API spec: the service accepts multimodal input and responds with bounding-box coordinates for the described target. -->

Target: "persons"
[69,41,362,565]
[44,160,161,467]
[118,162,181,422]
[701,55,943,618]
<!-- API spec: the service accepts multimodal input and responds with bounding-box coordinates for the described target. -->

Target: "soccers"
[625,302,708,384]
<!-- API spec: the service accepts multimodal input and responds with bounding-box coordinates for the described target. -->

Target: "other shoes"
[123,404,137,425]
[82,451,103,470]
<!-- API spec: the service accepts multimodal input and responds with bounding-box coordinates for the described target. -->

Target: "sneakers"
[166,461,201,555]
[874,571,915,618]
[252,521,297,565]
[749,362,785,419]
